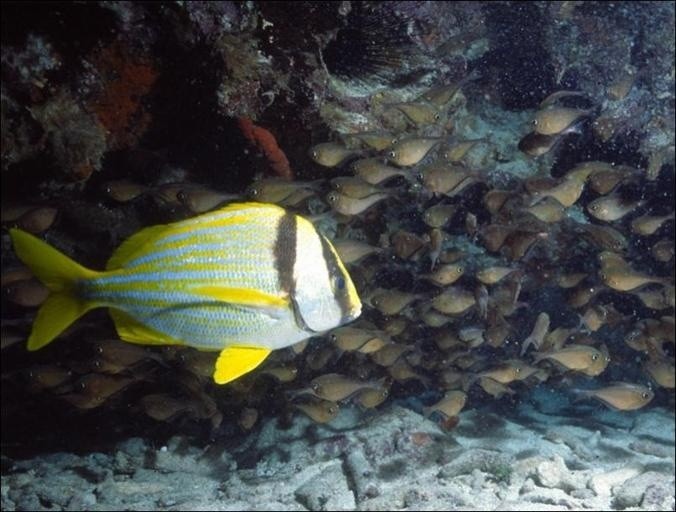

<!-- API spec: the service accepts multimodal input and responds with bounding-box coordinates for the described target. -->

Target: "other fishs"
[0,67,676,431]
